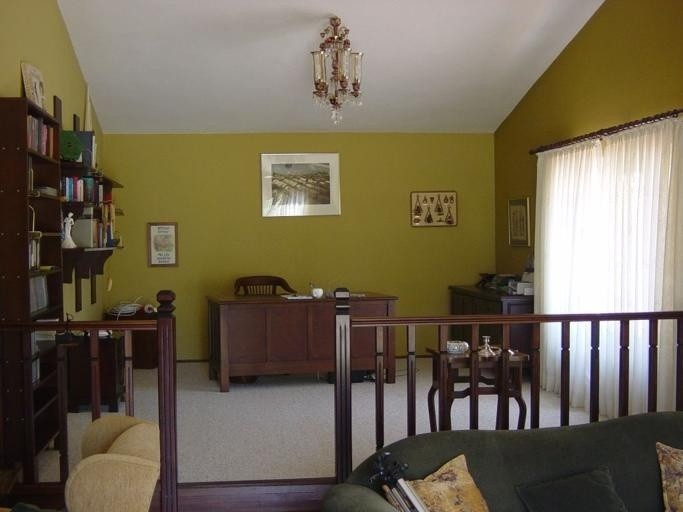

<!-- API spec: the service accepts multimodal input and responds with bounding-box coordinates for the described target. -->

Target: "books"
[381,478,430,512]
[27,114,57,312]
[61,176,115,248]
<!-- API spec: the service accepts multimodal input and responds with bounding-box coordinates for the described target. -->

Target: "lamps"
[304,16,366,128]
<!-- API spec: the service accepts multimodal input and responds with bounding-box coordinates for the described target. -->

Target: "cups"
[311,288,323,300]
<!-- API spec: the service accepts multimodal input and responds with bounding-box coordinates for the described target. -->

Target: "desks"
[202,288,402,393]
[422,345,529,432]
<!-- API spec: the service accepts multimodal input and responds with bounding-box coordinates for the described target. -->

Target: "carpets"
[23,359,614,485]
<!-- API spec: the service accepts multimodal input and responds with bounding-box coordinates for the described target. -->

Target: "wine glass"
[477,335,495,357]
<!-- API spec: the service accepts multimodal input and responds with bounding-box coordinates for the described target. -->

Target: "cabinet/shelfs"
[446,283,535,380]
[59,153,125,311]
[97,306,161,371]
[0,95,71,473]
[35,327,129,415]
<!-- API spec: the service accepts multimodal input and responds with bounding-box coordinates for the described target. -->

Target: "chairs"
[11,412,163,511]
[227,272,301,296]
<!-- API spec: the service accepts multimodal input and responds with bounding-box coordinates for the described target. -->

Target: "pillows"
[650,437,682,510]
[405,450,491,512]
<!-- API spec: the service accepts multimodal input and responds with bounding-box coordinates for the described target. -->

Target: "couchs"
[315,408,682,511]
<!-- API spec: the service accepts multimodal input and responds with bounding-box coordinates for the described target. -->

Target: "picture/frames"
[145,221,178,268]
[503,194,532,249]
[408,189,460,230]
[257,150,343,220]
